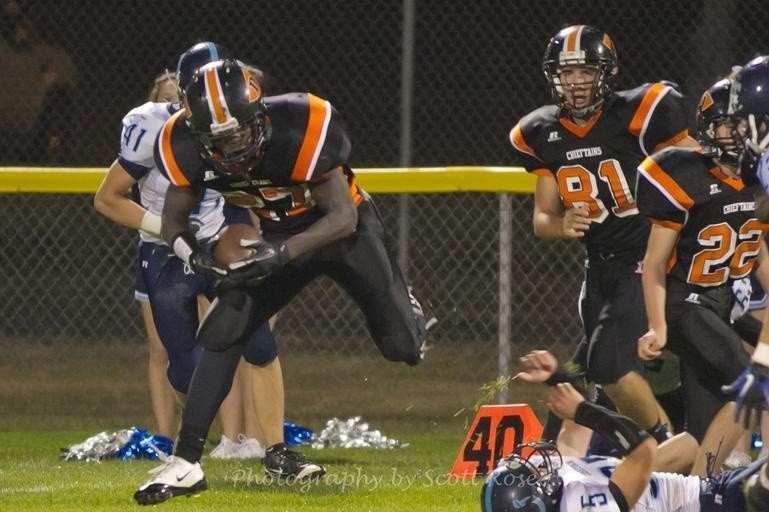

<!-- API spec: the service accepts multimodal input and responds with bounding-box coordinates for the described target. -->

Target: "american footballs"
[211,222,259,269]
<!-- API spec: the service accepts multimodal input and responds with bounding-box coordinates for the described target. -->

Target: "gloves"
[722,343,769,428]
[173,234,244,290]
[227,238,290,286]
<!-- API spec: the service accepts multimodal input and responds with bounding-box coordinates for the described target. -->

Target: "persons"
[480,23,769,512]
[134,72,182,439]
[36,63,81,163]
[198,295,276,457]
[94,39,328,483]
[131,57,427,504]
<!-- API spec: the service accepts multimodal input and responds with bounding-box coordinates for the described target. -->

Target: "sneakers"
[404,287,429,367]
[134,454,208,505]
[209,434,265,459]
[261,444,326,483]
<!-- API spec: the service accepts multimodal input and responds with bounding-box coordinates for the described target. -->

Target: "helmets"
[542,25,619,119]
[479,440,565,511]
[184,60,273,179]
[176,41,231,105]
[694,54,768,181]
